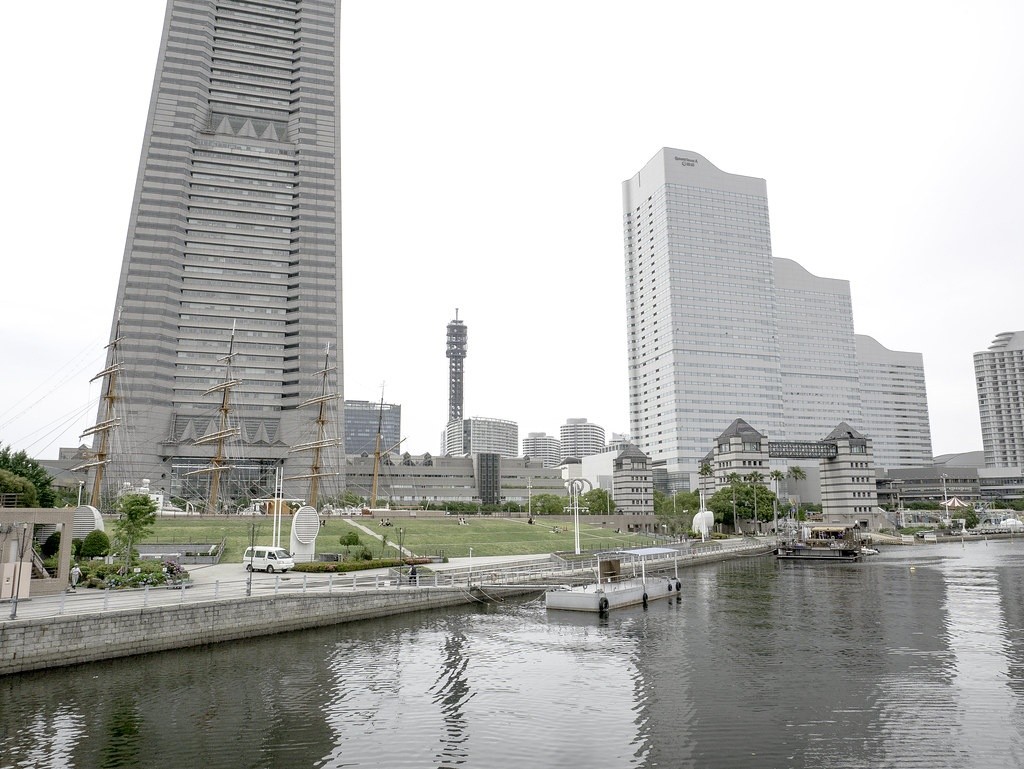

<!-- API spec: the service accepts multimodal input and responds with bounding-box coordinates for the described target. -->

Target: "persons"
[379,518,394,527]
[69,563,83,589]
[320,520,325,526]
[528,517,559,532]
[458,518,465,525]
[407,562,416,585]
[615,528,622,534]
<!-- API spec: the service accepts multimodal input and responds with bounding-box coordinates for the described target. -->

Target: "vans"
[243,545,295,573]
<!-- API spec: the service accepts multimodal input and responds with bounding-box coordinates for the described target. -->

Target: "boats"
[773,524,873,564]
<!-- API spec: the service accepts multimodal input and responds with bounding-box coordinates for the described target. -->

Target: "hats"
[75,563,78,565]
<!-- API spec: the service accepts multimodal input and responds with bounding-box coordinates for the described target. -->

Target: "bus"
[974,509,1019,526]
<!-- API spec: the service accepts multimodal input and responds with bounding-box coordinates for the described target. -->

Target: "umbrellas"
[939,494,968,509]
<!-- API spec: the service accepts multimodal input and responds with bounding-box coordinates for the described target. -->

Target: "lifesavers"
[643,592,648,600]
[676,582,681,591]
[599,597,609,611]
[668,584,672,590]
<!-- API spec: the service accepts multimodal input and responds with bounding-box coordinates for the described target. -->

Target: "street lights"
[940,473,950,519]
